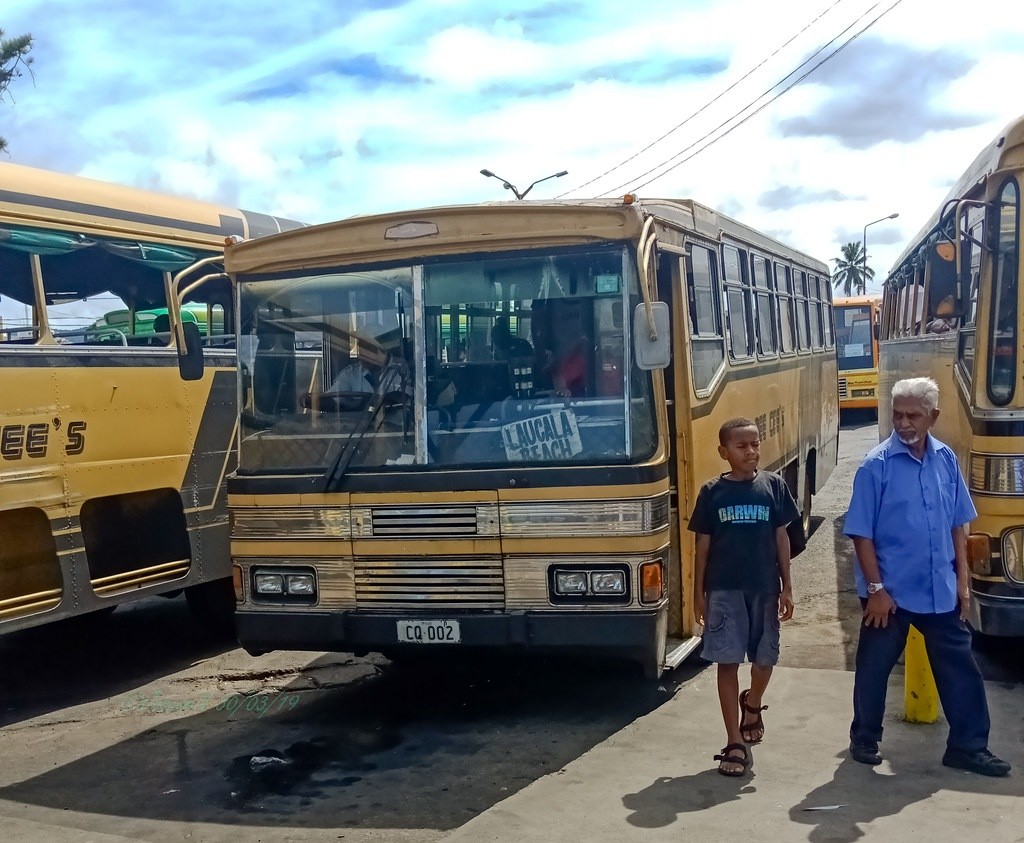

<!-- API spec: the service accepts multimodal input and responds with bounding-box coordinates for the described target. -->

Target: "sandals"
[737,688,769,744]
[713,743,748,779]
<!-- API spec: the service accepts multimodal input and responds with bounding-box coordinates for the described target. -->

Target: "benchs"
[0,325,234,348]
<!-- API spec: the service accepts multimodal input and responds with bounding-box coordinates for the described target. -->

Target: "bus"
[872,114,1024,640]
[167,194,836,684]
[0,164,413,640]
[831,297,884,420]
[83,299,519,349]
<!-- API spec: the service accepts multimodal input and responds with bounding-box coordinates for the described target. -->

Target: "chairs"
[837,324,870,347]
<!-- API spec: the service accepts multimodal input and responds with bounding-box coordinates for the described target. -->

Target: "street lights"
[863,213,902,295]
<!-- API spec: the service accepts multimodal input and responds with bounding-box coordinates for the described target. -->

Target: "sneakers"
[941,749,1011,778]
[848,738,882,765]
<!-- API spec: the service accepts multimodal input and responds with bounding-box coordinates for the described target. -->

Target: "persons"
[301,322,413,408]
[150,314,170,348]
[457,348,467,368]
[686,417,802,778]
[487,317,532,362]
[551,313,625,399]
[842,376,1014,778]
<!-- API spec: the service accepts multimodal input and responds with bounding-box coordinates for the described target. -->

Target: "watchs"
[867,582,884,594]
